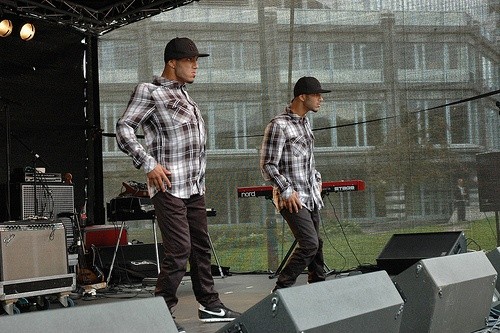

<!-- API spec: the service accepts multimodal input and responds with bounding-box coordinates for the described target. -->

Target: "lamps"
[0,13,40,46]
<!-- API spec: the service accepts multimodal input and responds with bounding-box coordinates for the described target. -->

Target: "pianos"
[106,196,225,284]
[237,179,365,279]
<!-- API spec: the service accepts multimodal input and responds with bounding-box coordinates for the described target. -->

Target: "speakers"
[214,270,403,333]
[0,295,178,333]
[376,230,468,276]
[486,247,500,307]
[389,251,497,333]
[0,220,68,281]
[19,181,76,238]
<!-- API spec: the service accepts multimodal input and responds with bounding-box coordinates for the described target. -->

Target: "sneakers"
[198,305,242,322]
[172,315,186,333]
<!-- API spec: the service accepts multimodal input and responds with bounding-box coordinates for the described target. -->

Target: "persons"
[113,37,247,333]
[260,76,337,294]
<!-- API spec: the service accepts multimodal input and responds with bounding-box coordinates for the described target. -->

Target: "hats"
[294,76,333,98]
[164,37,210,63]
